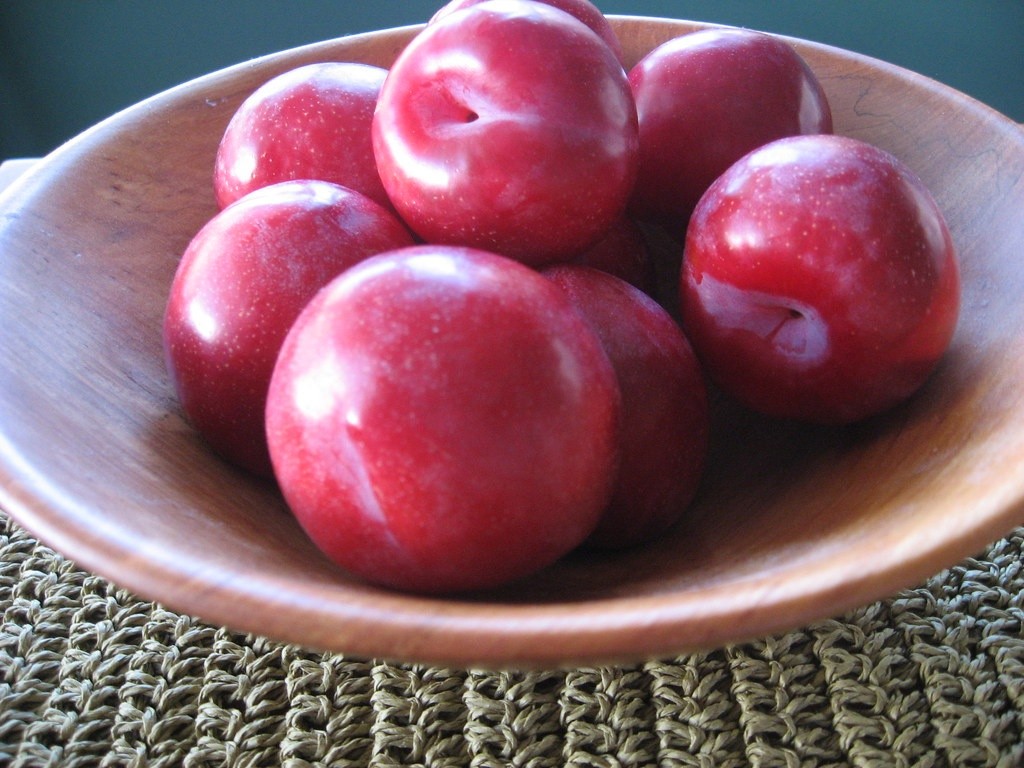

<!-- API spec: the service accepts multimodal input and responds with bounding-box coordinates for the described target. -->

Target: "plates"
[0,0,1024,674]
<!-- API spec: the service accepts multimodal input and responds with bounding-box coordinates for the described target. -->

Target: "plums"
[163,0,960,598]
